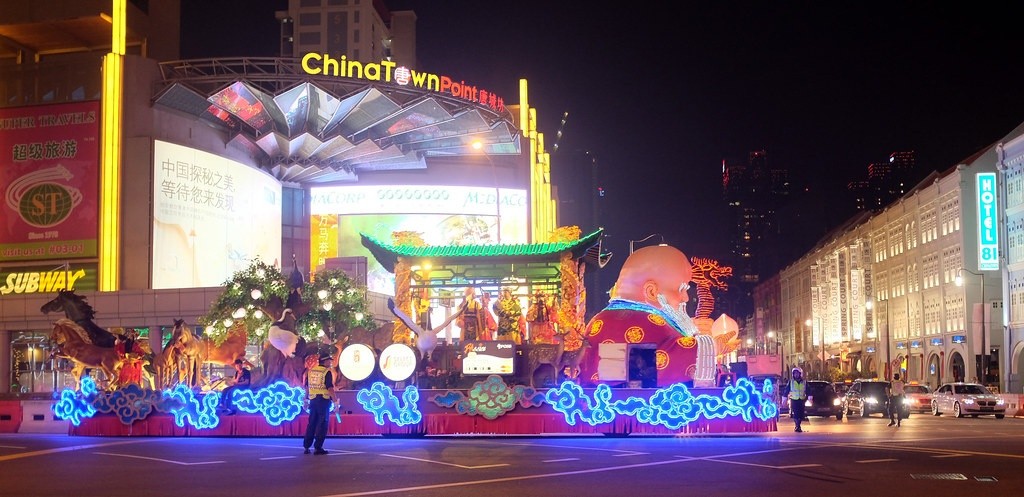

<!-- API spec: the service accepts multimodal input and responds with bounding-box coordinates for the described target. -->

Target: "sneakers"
[313,448,329,455]
[794,426,803,432]
[895,420,902,428]
[887,418,896,427]
[304,447,312,455]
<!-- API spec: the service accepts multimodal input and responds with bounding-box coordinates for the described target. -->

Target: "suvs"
[749,373,789,414]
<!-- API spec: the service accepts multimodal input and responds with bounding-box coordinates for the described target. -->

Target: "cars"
[903,380,933,415]
[831,380,852,399]
[931,382,1005,419]
[782,382,843,420]
[843,377,909,419]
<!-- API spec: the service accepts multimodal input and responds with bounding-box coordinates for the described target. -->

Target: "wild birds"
[267,251,308,379]
[386,295,469,376]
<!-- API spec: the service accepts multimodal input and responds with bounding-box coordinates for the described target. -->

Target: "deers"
[526,326,594,391]
[301,334,350,389]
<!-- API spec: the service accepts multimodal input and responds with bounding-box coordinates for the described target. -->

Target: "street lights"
[767,330,784,377]
[805,315,825,374]
[955,268,985,387]
[865,297,889,381]
[748,339,760,355]
[628,233,668,255]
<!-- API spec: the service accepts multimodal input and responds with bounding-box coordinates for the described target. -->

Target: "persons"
[458,287,558,346]
[303,353,339,456]
[411,364,462,390]
[785,367,809,432]
[215,359,250,415]
[885,374,906,427]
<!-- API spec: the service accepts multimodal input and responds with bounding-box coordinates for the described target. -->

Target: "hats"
[791,367,804,380]
[893,374,901,380]
[316,352,334,362]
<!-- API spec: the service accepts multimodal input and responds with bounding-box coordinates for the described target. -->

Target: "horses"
[39,287,257,402]
